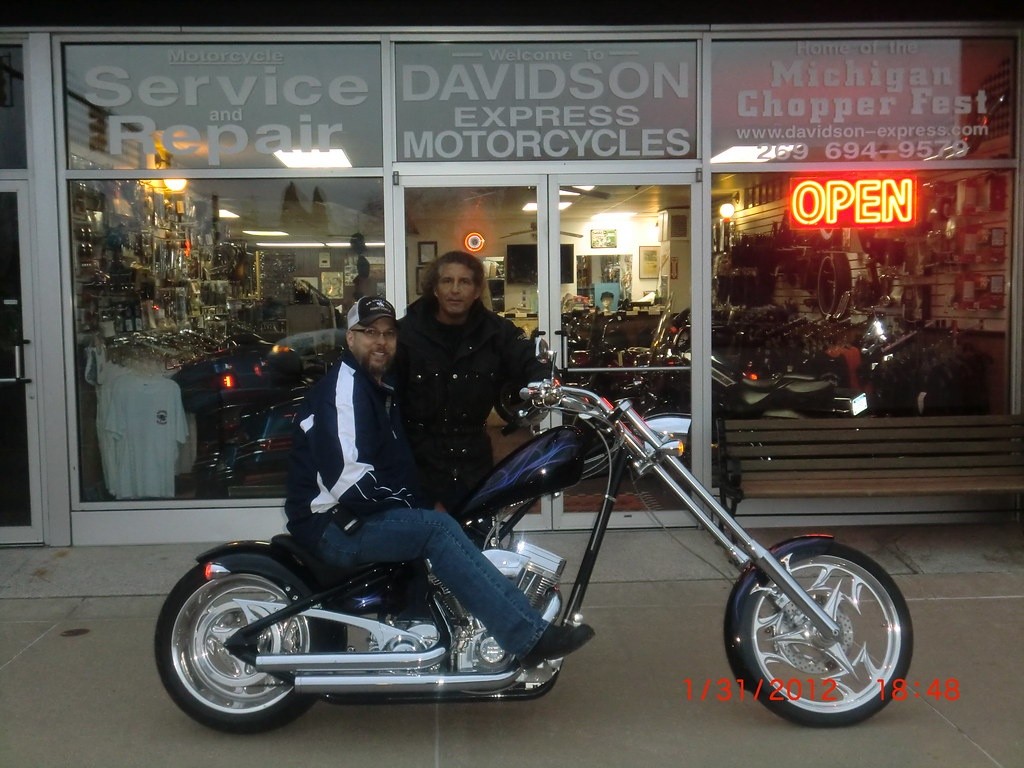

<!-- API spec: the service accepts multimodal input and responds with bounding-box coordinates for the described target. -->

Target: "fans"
[501,222,584,239]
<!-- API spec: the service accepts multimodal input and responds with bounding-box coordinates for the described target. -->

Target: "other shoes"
[519,623,596,673]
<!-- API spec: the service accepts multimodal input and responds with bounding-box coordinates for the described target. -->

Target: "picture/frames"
[639,246,660,278]
[416,241,438,294]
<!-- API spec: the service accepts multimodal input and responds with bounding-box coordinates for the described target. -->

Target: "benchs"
[711,416,1024,561]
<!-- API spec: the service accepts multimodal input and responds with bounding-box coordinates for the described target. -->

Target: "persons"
[282,296,597,666]
[600,291,615,312]
[382,249,561,510]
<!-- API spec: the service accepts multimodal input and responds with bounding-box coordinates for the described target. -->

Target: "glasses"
[351,328,398,336]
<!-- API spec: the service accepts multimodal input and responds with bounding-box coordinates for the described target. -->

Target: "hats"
[347,295,396,330]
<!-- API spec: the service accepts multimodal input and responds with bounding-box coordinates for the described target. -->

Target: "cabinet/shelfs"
[487,277,505,313]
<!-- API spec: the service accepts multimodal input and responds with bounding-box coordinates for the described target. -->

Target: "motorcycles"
[181,339,313,501]
[149,302,919,729]
[495,305,872,486]
